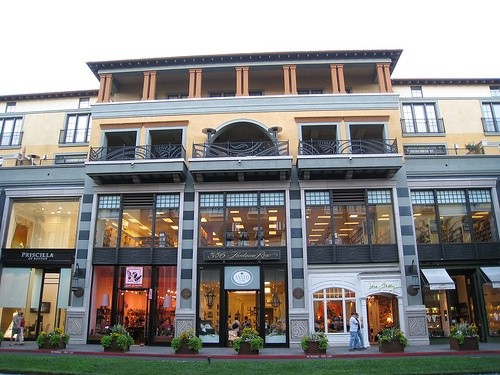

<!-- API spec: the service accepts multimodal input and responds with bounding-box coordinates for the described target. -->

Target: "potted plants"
[101,325,135,352]
[378,328,408,352]
[449,321,480,350]
[233,327,264,355]
[171,330,202,354]
[37,328,69,350]
[301,332,329,355]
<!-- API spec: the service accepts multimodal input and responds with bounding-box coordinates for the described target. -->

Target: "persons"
[9,311,27,346]
[313,312,343,334]
[348,312,367,351]
[226,225,264,246]
[232,315,252,339]
[96,309,175,337]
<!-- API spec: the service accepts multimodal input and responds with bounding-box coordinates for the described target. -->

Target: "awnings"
[421,269,457,290]
[480,267,500,288]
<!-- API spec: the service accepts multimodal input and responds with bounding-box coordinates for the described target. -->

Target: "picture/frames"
[30,302,50,313]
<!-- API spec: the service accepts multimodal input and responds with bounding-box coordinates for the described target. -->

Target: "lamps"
[408,260,421,291]
[163,296,171,311]
[271,271,280,310]
[100,294,109,318]
[204,272,216,309]
[202,128,216,142]
[268,126,282,140]
[72,263,82,295]
